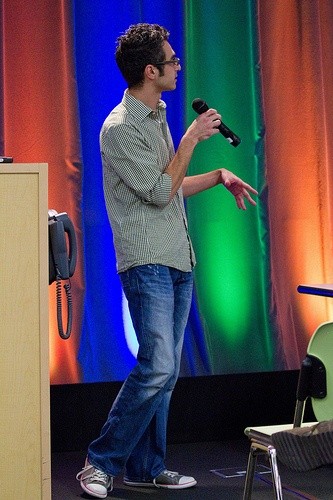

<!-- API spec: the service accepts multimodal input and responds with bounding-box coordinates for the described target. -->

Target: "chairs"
[242,321,333,500]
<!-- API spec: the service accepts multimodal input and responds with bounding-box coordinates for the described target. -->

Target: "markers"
[0,156,13,163]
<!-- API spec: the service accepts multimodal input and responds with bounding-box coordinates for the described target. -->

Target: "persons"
[76,23,258,498]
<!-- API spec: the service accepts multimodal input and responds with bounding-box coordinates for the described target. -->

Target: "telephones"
[48,208,78,340]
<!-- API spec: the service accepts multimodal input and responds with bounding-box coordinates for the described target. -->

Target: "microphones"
[192,98,241,147]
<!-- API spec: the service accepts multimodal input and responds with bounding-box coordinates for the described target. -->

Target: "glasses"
[153,57,180,66]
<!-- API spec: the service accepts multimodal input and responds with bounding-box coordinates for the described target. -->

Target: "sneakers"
[123,469,197,489]
[75,455,113,498]
